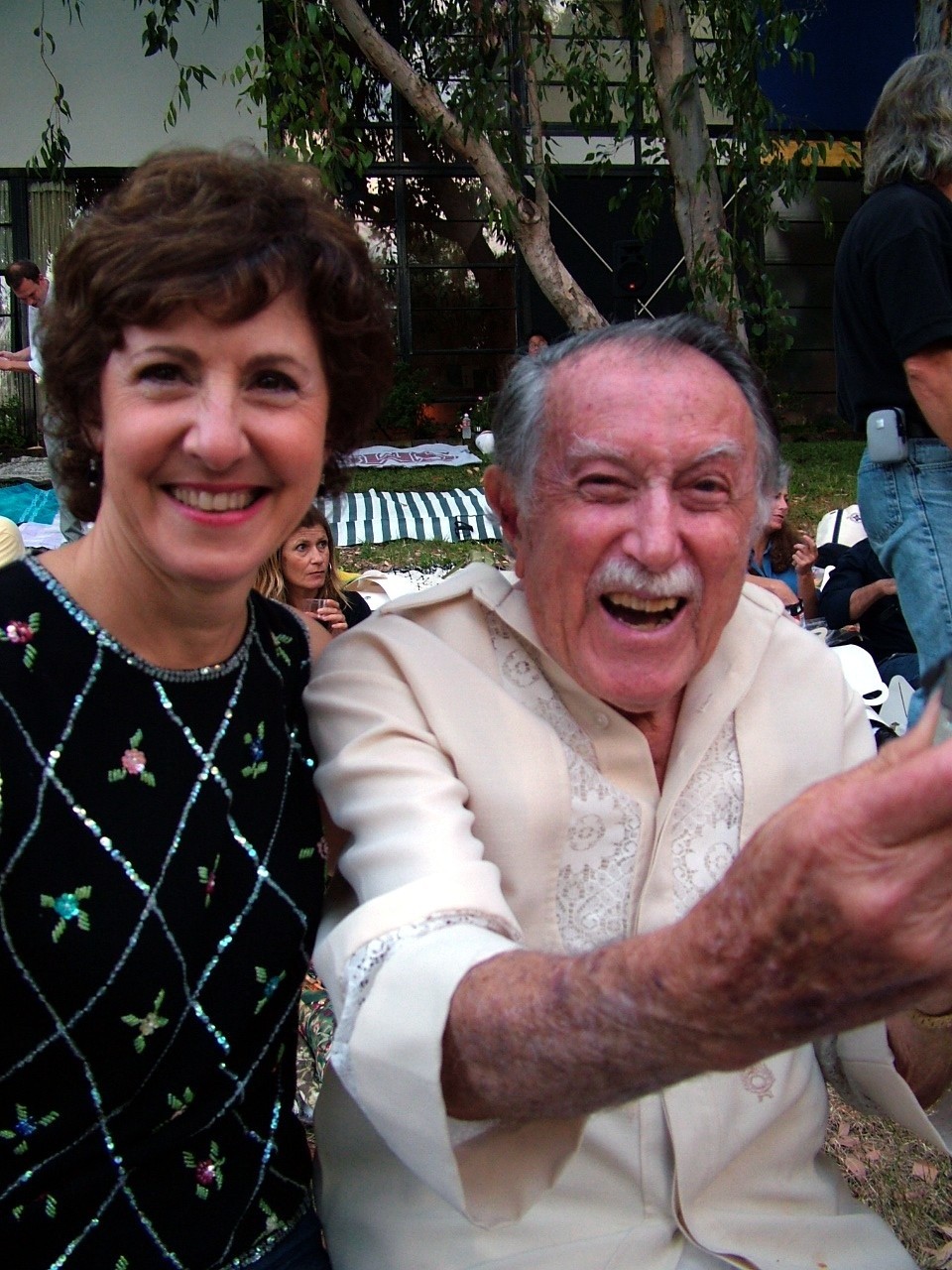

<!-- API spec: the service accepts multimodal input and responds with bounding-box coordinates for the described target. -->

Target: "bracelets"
[905,1008,952,1030]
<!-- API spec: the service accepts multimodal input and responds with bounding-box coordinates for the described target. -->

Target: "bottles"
[462,413,471,439]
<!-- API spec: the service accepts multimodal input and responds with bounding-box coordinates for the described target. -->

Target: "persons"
[744,484,818,622]
[842,51,952,719]
[527,334,550,355]
[820,537,921,692]
[255,505,372,638]
[0,142,358,1270]
[0,260,54,380]
[303,313,952,1270]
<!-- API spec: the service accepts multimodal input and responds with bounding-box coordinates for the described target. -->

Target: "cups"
[304,599,331,631]
